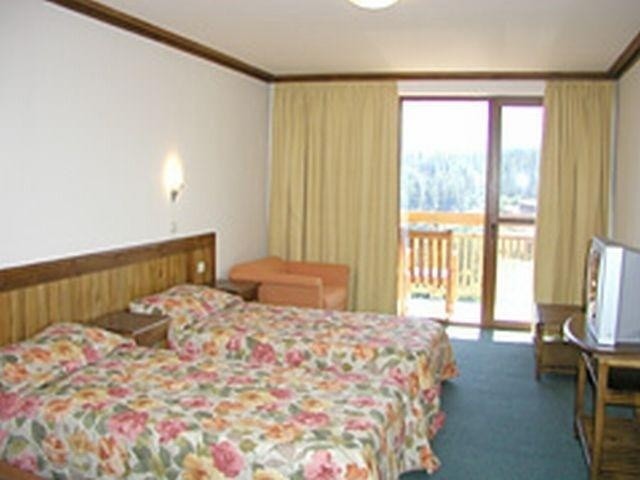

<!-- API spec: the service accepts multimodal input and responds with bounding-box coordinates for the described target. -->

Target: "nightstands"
[95,311,172,349]
[218,277,262,302]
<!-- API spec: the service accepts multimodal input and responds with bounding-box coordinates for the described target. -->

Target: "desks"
[534,302,585,383]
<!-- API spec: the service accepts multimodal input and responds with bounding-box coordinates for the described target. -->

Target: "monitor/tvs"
[585,236,640,346]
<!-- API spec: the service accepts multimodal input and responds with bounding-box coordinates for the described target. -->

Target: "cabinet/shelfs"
[563,313,640,480]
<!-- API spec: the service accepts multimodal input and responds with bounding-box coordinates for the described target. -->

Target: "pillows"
[127,284,243,327]
[0,321,136,415]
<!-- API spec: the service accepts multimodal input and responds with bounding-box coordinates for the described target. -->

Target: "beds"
[0,318,441,480]
[132,283,460,406]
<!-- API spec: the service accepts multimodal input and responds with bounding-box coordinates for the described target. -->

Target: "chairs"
[228,254,351,311]
[405,231,459,311]
